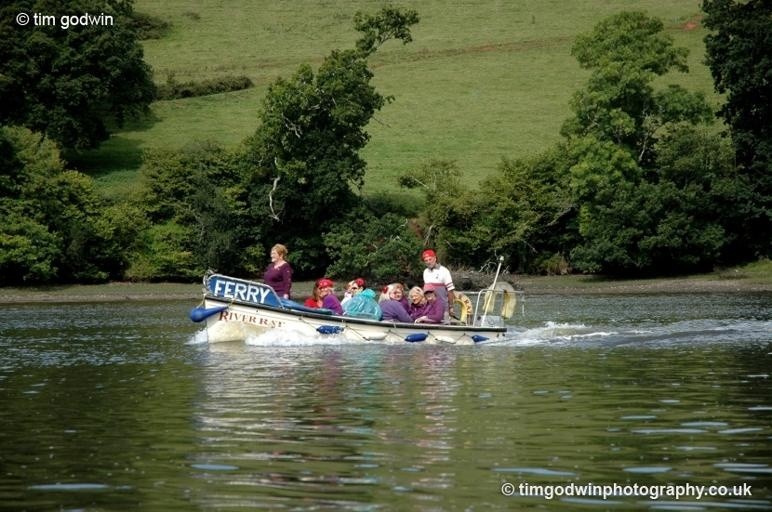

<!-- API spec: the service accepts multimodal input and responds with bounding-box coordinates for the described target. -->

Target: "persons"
[263,242,456,323]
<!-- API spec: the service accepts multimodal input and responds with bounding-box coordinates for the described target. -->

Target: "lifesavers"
[450,290,473,323]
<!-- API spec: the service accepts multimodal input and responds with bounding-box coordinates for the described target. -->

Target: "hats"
[355,278,368,289]
[423,284,436,295]
[422,249,436,257]
[319,280,333,289]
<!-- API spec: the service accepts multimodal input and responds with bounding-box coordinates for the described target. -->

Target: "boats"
[198,267,511,349]
